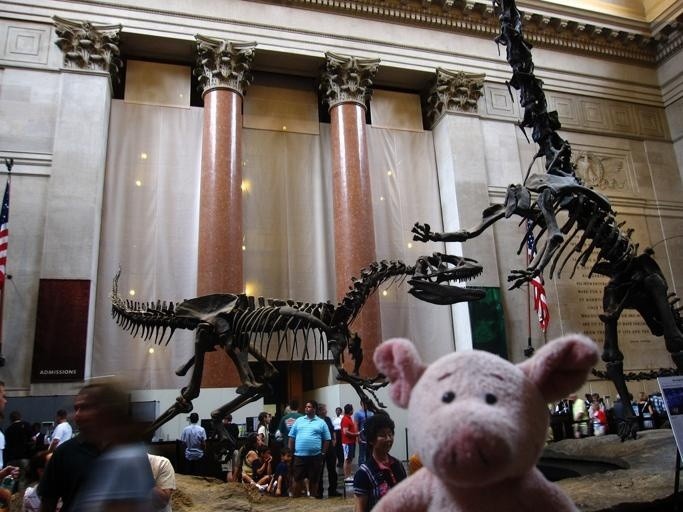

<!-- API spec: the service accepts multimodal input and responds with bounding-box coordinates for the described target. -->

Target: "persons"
[257,412,271,446]
[0,466,19,483]
[148,452,173,511]
[341,402,361,483]
[266,447,291,495]
[239,432,261,477]
[181,412,208,461]
[588,393,606,436]
[354,398,379,466]
[613,394,623,418]
[289,401,330,500]
[638,391,653,428]
[39,384,110,512]
[315,403,343,499]
[268,400,290,446]
[354,410,406,512]
[48,408,73,452]
[332,406,345,468]
[0,381,20,512]
[4,410,39,466]
[22,450,63,512]
[211,413,239,482]
[243,446,272,492]
[569,393,589,438]
[275,400,303,447]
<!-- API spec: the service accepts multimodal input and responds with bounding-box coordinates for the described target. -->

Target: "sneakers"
[344,478,354,486]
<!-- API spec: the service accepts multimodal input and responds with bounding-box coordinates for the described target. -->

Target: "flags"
[523,214,553,334]
[0,159,13,282]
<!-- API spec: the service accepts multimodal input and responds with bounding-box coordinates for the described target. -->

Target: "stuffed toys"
[369,332,599,512]
